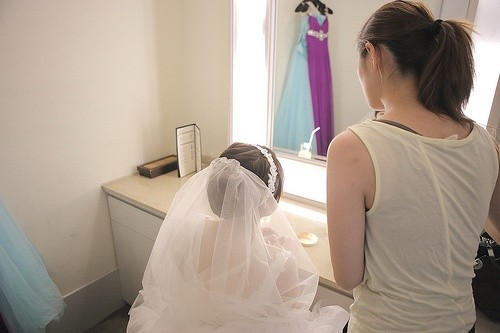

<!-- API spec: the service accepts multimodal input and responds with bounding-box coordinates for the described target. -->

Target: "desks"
[101,155,353,313]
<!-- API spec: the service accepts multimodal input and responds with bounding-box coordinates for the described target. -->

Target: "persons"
[326,0,500,333]
[127,142,350,332]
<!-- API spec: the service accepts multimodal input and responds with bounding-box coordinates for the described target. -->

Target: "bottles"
[298,142,311,158]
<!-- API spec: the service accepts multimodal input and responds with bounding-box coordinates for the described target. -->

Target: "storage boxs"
[137,154,178,178]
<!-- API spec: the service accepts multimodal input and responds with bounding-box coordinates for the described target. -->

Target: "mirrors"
[267,0,391,168]
